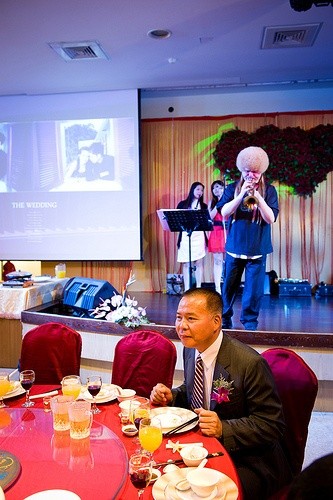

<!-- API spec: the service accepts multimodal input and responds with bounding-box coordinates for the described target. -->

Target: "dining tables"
[0,382,243,500]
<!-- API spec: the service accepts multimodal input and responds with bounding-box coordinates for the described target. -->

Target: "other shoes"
[222,318,232,328]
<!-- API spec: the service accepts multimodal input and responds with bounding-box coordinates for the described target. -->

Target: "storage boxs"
[0,277,70,319]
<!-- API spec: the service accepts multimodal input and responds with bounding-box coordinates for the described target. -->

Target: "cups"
[43,397,52,412]
[55,264,66,279]
[62,375,81,399]
[51,431,91,471]
[49,395,76,431]
[68,400,91,439]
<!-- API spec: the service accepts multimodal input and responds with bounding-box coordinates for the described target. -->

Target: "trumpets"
[244,178,259,209]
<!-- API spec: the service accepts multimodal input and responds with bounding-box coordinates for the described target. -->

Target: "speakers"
[61,276,120,311]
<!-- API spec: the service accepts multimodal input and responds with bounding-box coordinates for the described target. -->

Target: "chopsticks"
[165,416,199,436]
[157,452,224,466]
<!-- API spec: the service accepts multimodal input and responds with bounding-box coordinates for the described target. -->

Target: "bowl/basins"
[186,468,219,497]
[180,445,208,467]
[139,467,161,485]
[120,400,141,436]
[116,389,136,403]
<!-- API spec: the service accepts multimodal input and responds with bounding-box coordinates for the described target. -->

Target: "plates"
[148,407,199,433]
[152,467,238,500]
[80,383,122,403]
[24,489,81,500]
[0,380,26,400]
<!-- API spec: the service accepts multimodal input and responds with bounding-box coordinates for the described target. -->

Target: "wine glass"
[130,397,151,445]
[128,455,152,500]
[139,416,162,481]
[19,370,35,408]
[0,376,11,408]
[86,376,102,414]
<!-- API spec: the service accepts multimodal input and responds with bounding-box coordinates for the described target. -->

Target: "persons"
[208,180,228,296]
[216,146,281,332]
[175,182,212,292]
[65,141,115,182]
[149,288,295,500]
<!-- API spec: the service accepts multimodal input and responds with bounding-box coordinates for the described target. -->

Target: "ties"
[191,356,204,413]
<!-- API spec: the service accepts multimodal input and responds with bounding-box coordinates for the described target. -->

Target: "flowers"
[210,373,234,403]
[214,123,333,199]
[89,269,156,328]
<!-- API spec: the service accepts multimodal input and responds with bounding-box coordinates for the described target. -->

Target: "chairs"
[18,321,82,384]
[108,330,177,400]
[259,348,318,500]
